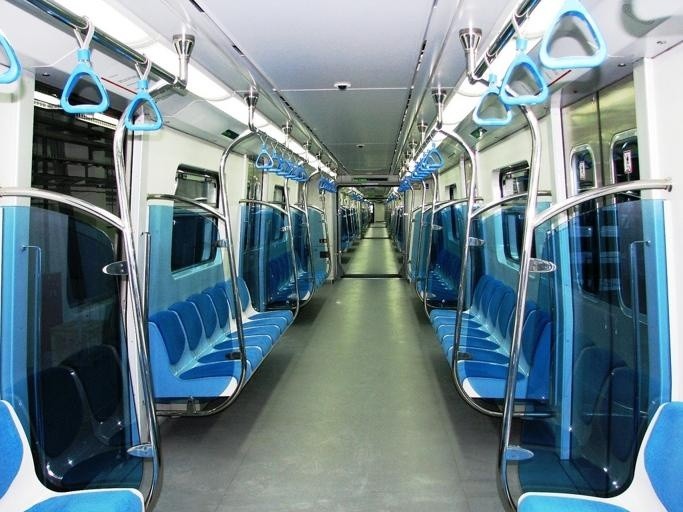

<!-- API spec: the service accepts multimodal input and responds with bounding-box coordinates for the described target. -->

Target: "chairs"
[148,251,328,424]
[424,247,554,462]
[0,399,148,511]
[515,399,683,512]
